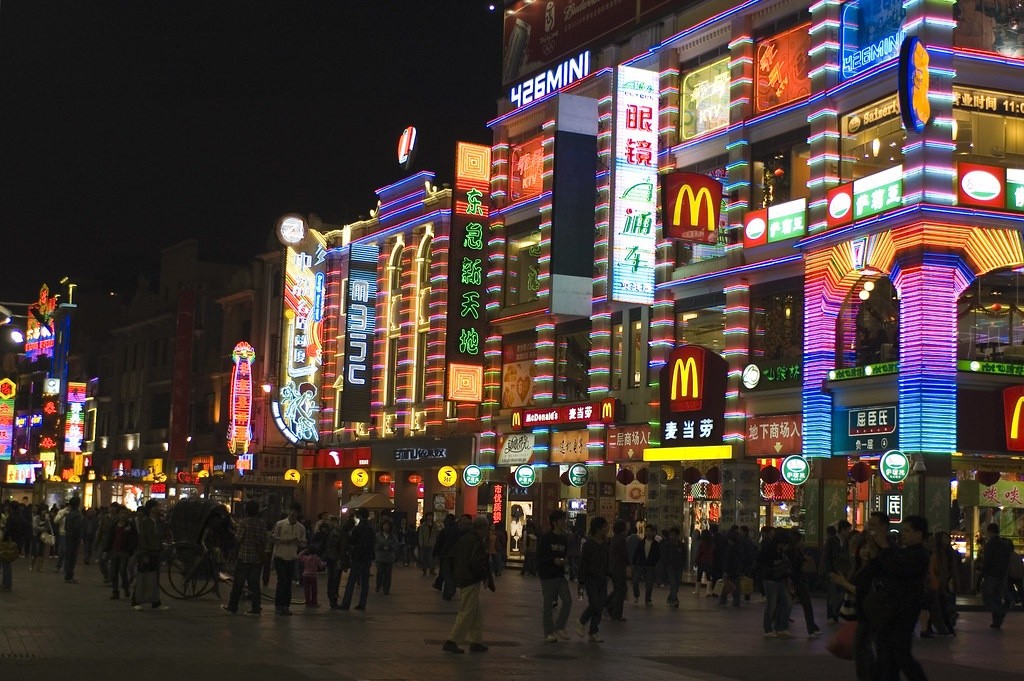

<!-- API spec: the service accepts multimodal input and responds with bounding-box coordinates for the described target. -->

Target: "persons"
[0,490,509,616]
[441,517,496,654]
[520,512,1024,681]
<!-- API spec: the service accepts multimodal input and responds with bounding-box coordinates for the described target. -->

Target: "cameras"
[868,530,876,536]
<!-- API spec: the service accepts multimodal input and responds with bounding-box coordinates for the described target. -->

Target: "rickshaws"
[157,499,308,605]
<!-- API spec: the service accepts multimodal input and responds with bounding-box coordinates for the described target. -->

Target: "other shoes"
[645,602,653,608]
[0,584,11,591]
[588,634,604,642]
[224,588,390,611]
[990,623,1000,628]
[826,618,835,624]
[469,643,488,651]
[83,560,169,612]
[545,634,557,642]
[764,629,793,639]
[575,618,587,637]
[64,576,77,583]
[556,629,569,640]
[442,642,464,654]
[612,616,627,621]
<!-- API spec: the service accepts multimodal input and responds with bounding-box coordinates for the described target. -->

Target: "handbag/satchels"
[984,576,999,588]
[740,576,753,594]
[40,531,55,546]
[712,577,737,596]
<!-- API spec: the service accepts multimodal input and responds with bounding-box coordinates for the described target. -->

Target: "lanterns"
[683,467,701,484]
[706,466,721,485]
[408,474,422,483]
[561,471,572,486]
[850,462,872,484]
[636,467,648,484]
[335,480,343,490]
[760,465,780,484]
[976,471,1000,487]
[617,469,634,485]
[379,475,392,484]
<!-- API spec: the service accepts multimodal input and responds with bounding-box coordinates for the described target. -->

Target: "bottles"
[578,582,583,600]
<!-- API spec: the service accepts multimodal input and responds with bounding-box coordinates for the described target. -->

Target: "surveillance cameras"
[913,461,926,475]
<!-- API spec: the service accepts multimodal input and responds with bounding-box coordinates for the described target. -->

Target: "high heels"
[809,631,823,637]
